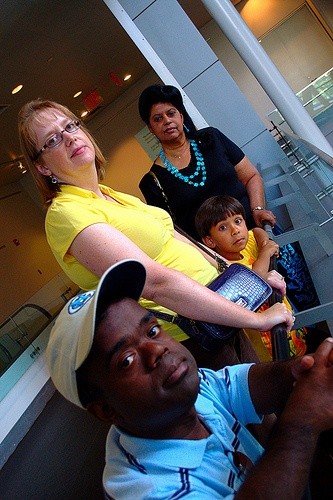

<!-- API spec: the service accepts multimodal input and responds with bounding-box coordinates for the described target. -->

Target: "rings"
[281,276,285,280]
[292,316,296,323]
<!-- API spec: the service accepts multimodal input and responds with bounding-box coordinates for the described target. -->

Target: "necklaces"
[164,142,188,159]
[103,193,108,200]
[159,139,207,187]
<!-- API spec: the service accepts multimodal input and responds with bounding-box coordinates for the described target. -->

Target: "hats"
[46,259,146,411]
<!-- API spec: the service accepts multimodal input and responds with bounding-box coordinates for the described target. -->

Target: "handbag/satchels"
[172,262,273,353]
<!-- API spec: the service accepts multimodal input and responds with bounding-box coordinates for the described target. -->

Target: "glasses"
[33,119,81,161]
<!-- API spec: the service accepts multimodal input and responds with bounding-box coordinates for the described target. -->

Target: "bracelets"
[252,206,265,213]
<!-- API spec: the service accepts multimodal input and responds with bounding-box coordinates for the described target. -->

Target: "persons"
[196,195,308,361]
[18,100,296,438]
[138,84,320,329]
[46,258,333,500]
